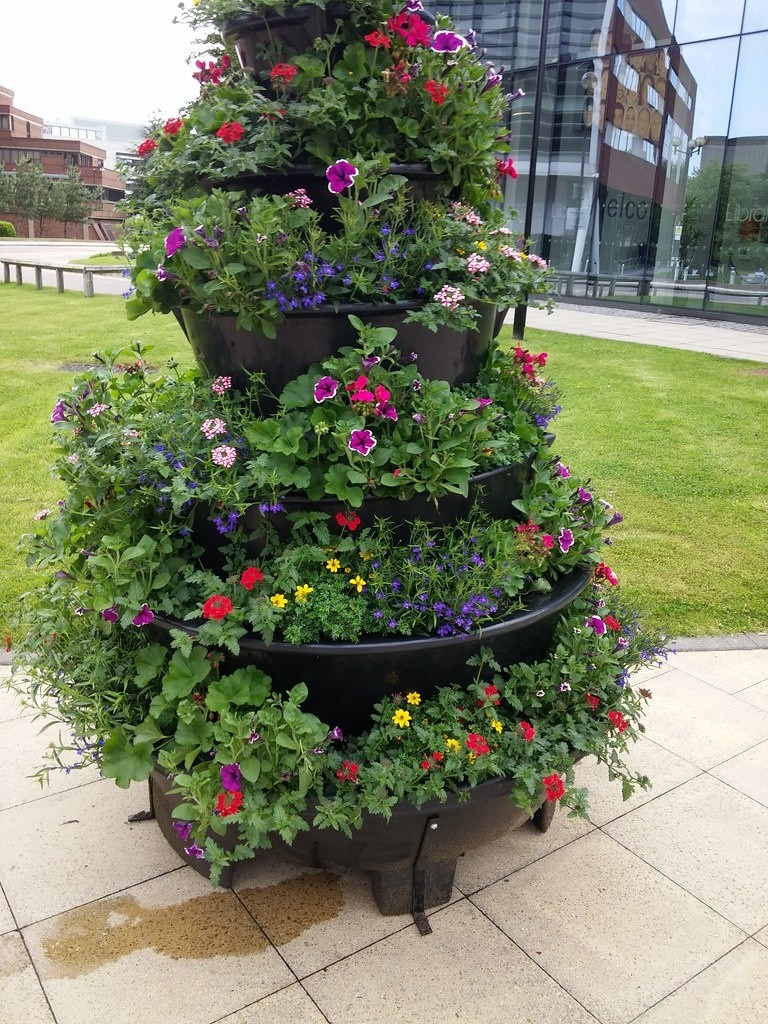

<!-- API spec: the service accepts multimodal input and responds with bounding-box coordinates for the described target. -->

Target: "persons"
[682,262,708,278]
[755,267,765,277]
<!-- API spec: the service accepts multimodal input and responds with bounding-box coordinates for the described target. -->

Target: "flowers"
[17,1,638,889]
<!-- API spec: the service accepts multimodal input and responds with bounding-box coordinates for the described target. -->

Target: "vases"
[124,158,593,917]
